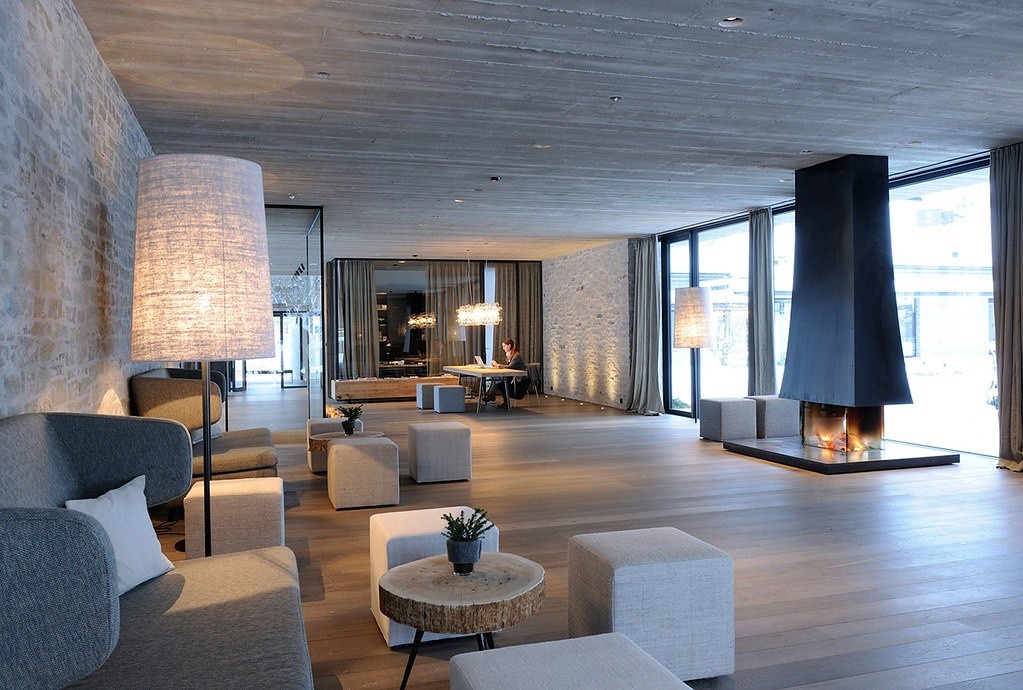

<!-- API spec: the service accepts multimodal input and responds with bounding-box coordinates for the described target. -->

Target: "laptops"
[474,356,496,369]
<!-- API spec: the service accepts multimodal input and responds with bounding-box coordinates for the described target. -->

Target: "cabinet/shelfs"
[331,375,460,403]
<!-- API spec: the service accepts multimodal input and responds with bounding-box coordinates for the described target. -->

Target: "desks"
[444,366,528,414]
[394,358,420,378]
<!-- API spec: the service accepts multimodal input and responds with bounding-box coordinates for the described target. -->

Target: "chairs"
[511,362,541,408]
[0,412,316,690]
[131,367,278,523]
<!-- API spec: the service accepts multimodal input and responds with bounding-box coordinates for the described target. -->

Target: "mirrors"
[334,257,487,396]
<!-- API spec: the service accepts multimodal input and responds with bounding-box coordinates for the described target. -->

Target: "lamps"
[672,287,716,428]
[454,242,502,327]
[130,152,275,558]
[405,311,438,330]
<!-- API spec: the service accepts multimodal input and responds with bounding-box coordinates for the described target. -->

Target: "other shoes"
[482,397,496,402]
[498,402,510,407]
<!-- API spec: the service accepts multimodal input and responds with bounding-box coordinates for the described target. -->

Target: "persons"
[482,340,526,408]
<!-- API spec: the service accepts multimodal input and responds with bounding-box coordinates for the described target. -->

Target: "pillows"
[66,474,176,598]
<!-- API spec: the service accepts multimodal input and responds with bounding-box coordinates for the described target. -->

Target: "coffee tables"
[309,431,385,454]
[379,552,546,690]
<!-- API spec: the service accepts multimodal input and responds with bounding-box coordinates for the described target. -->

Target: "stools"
[417,383,444,409]
[743,395,800,438]
[700,398,757,444]
[306,417,364,475]
[369,506,500,652]
[182,475,284,558]
[433,385,465,414]
[408,422,471,486]
[327,438,399,511]
[448,631,695,690]
[567,527,735,681]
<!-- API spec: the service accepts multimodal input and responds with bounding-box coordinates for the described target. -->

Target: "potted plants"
[338,404,364,437]
[440,508,495,576]
